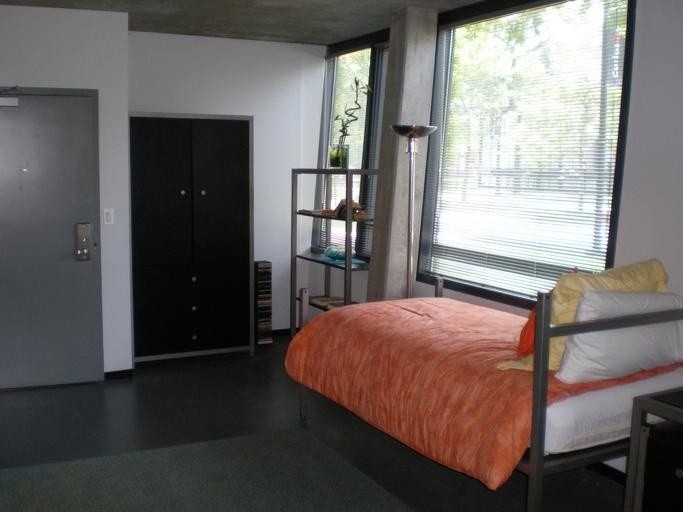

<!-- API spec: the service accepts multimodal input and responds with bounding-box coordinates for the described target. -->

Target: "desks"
[623,386,682,511]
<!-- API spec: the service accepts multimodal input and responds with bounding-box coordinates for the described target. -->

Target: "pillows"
[498,259,669,373]
[514,305,538,357]
[554,293,683,386]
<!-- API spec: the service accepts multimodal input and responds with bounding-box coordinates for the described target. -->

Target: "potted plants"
[329,76,371,169]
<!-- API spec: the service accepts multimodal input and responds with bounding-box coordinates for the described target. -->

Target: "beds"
[285,278,683,512]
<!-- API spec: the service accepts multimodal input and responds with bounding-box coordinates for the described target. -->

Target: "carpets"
[0,426,412,511]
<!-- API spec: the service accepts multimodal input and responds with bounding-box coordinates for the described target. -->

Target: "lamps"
[390,123,438,298]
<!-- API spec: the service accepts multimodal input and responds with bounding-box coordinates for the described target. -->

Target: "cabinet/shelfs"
[128,111,255,363]
[291,167,380,337]
